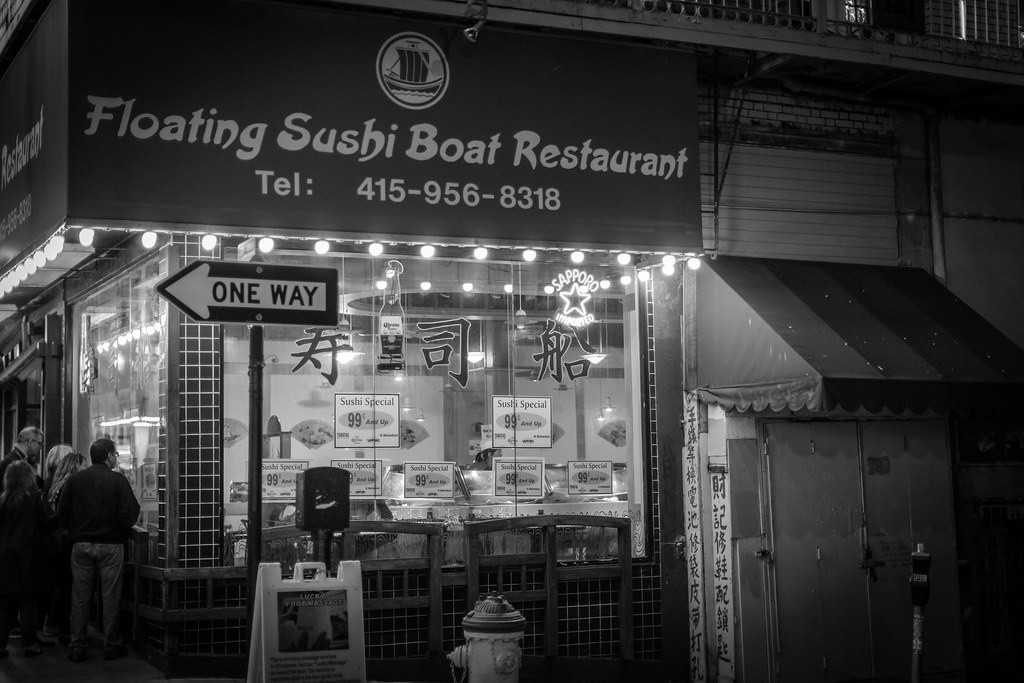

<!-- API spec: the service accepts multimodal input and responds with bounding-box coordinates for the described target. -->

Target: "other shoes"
[43,626,60,636]
[7,631,23,639]
[0,649,9,660]
[104,647,128,660]
[35,635,57,648]
[24,648,43,658]
[68,654,85,663]
[59,635,92,644]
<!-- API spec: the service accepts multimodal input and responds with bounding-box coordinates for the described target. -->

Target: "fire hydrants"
[447,591,528,682]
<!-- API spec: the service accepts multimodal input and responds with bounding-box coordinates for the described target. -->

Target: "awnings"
[698,255,1024,418]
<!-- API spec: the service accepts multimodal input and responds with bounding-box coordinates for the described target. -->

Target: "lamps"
[26,321,41,335]
[464,0,490,43]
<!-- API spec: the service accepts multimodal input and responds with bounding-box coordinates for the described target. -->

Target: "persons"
[0,426,87,659]
[55,439,141,662]
[468,448,502,471]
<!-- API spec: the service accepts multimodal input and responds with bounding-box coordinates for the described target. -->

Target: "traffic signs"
[153,259,339,331]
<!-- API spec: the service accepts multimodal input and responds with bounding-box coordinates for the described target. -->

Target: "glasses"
[111,452,119,458]
[31,440,43,448]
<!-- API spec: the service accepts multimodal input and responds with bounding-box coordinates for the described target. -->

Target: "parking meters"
[910,543,932,683]
